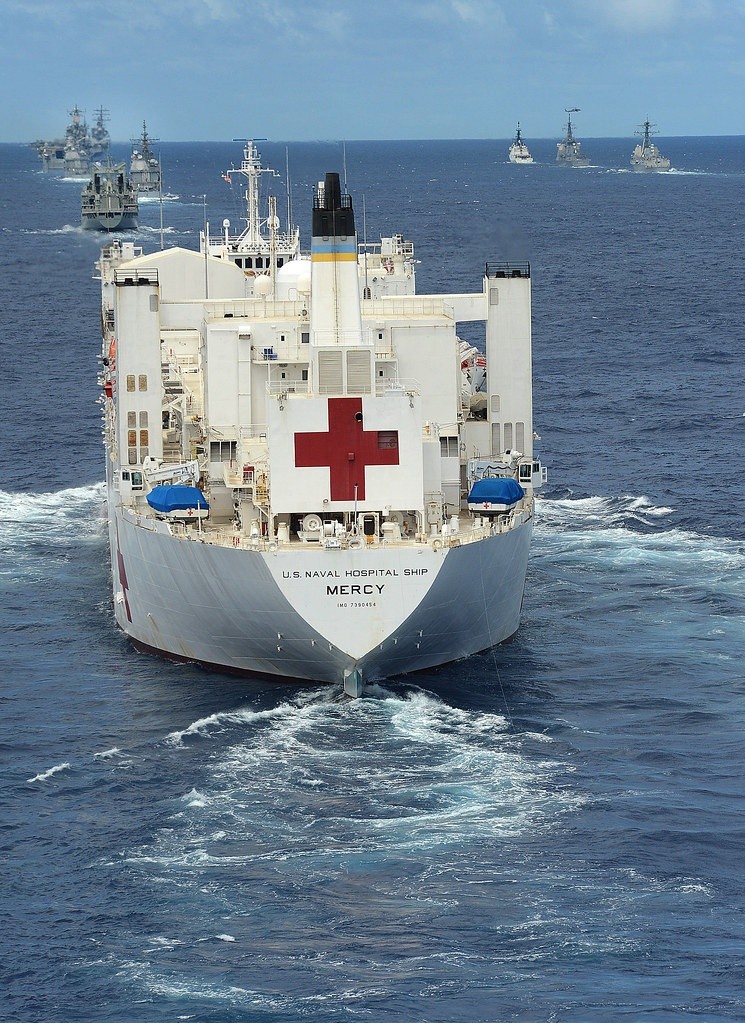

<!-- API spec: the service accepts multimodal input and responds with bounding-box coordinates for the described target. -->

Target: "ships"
[91,138,549,699]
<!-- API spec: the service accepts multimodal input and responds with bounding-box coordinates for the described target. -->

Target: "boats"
[79,140,141,231]
[507,121,534,164]
[554,106,593,167]
[630,113,671,171]
[129,120,164,196]
[27,104,113,178]
